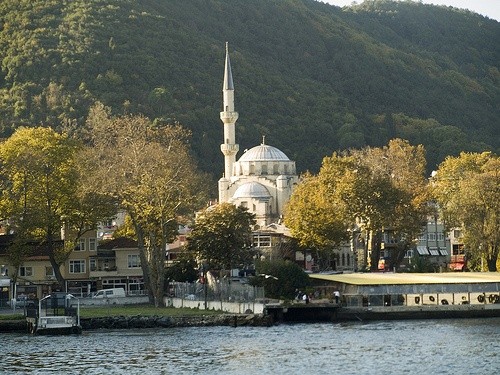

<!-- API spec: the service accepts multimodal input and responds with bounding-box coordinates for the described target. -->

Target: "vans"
[39,293,72,308]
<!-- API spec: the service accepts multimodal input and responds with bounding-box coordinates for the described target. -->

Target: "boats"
[303,268,500,319]
[25,293,83,335]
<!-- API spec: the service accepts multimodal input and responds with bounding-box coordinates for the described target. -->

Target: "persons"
[292,287,341,305]
[65,290,78,309]
[52,295,59,316]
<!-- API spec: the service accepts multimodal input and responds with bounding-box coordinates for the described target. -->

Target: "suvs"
[92,287,126,299]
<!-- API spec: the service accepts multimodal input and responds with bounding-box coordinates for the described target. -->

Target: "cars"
[6,293,35,307]
